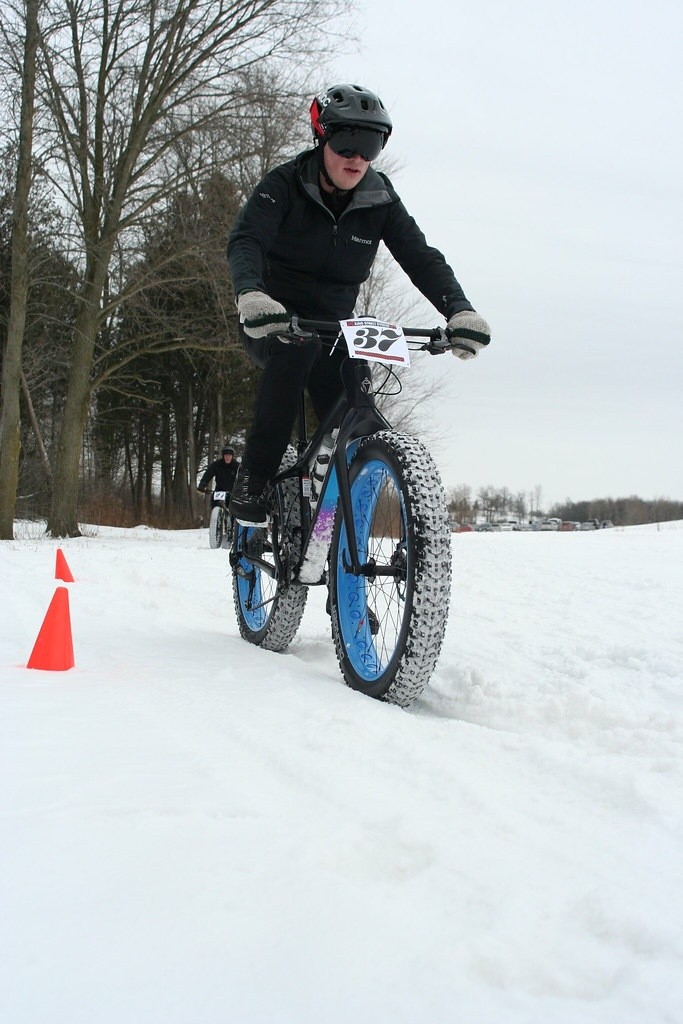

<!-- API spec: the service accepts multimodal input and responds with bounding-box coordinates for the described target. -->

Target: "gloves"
[238,291,290,339]
[198,487,204,493]
[446,310,491,361]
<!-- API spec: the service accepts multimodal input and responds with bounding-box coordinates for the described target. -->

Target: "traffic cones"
[25,587,75,671]
[54,549,75,583]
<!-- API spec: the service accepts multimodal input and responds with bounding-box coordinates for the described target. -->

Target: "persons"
[198,445,241,543]
[226,83,492,637]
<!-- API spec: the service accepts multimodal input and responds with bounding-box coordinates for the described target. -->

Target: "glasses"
[329,124,382,161]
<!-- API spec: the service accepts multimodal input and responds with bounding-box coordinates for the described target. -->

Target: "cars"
[450,517,611,533]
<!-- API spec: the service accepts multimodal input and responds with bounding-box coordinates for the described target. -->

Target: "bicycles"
[201,487,237,549]
[229,316,485,707]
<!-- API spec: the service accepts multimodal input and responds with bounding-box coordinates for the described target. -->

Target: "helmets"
[309,83,393,150]
[222,446,235,456]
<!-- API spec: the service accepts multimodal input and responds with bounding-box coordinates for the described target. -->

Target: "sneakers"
[326,584,379,634]
[229,462,270,522]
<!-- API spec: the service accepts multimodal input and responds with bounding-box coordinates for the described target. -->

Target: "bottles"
[311,428,342,494]
